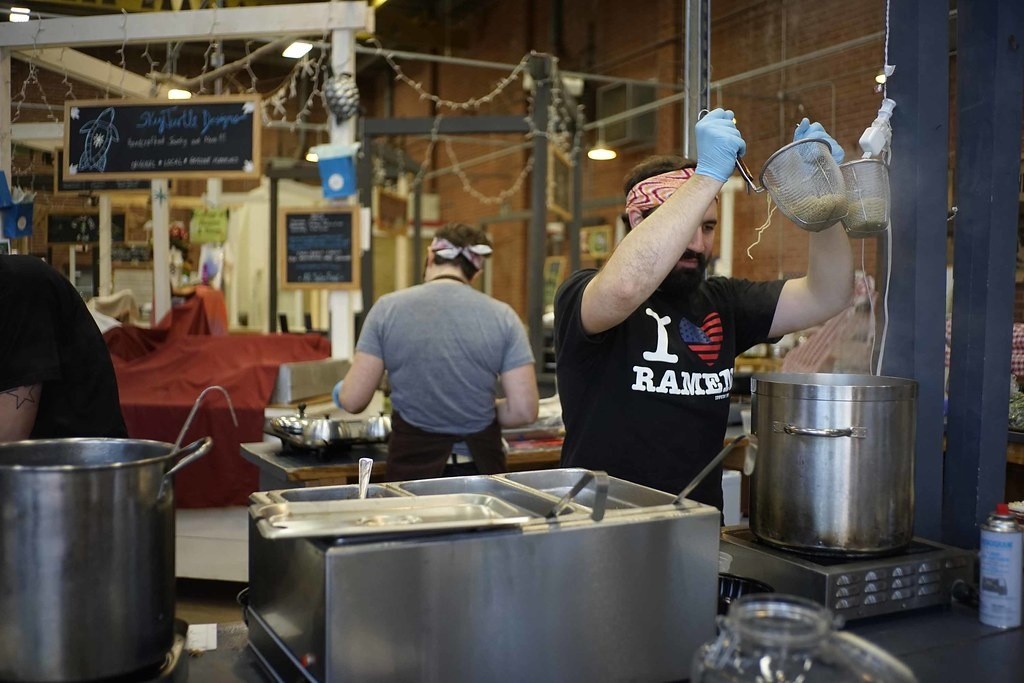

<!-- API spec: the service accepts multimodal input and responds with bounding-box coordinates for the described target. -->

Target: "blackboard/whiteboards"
[278,204,363,290]
[62,95,261,178]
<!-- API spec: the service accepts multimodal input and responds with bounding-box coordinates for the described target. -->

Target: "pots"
[750,372,917,557]
[0,436,213,683]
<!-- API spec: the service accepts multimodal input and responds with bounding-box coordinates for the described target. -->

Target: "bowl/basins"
[716,575,775,638]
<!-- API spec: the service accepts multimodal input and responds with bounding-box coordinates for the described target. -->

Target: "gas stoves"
[719,523,977,628]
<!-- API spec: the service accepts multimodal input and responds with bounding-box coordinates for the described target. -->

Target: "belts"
[445,454,473,464]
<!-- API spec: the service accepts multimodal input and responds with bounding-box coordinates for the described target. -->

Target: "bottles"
[689,592,917,683]
[979,503,1023,630]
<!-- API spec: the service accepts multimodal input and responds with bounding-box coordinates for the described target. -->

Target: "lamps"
[588,140,618,160]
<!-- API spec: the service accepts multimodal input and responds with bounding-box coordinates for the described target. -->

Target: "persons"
[0,248,134,443]
[555,107,844,525]
[324,219,538,480]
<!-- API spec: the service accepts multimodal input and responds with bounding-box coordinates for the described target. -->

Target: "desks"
[239,437,748,491]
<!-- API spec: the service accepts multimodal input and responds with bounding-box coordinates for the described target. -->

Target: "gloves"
[793,117,845,169]
[694,107,746,182]
